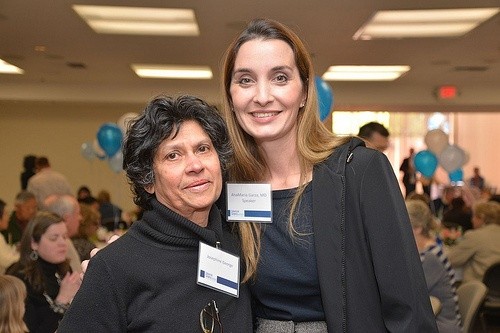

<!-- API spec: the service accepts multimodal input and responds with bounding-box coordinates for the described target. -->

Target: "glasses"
[200,300,223,333]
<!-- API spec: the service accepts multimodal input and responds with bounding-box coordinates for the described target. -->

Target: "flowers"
[440,226,466,238]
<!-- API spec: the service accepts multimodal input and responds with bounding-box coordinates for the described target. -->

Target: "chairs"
[429,259,500,333]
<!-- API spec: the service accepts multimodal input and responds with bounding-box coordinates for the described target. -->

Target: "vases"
[445,238,456,247]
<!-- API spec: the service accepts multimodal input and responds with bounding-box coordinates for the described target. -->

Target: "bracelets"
[55,301,69,311]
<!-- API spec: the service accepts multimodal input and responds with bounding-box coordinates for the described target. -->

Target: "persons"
[57,94,254,333]
[1,147,500,333]
[359,121,389,153]
[82,18,439,332]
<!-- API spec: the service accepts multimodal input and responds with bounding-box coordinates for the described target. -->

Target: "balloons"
[449,166,464,184]
[314,74,334,124]
[93,139,107,161]
[417,150,436,179]
[117,111,142,136]
[79,141,93,160]
[425,129,448,157]
[439,148,463,175]
[96,122,123,158]
[111,151,125,174]
